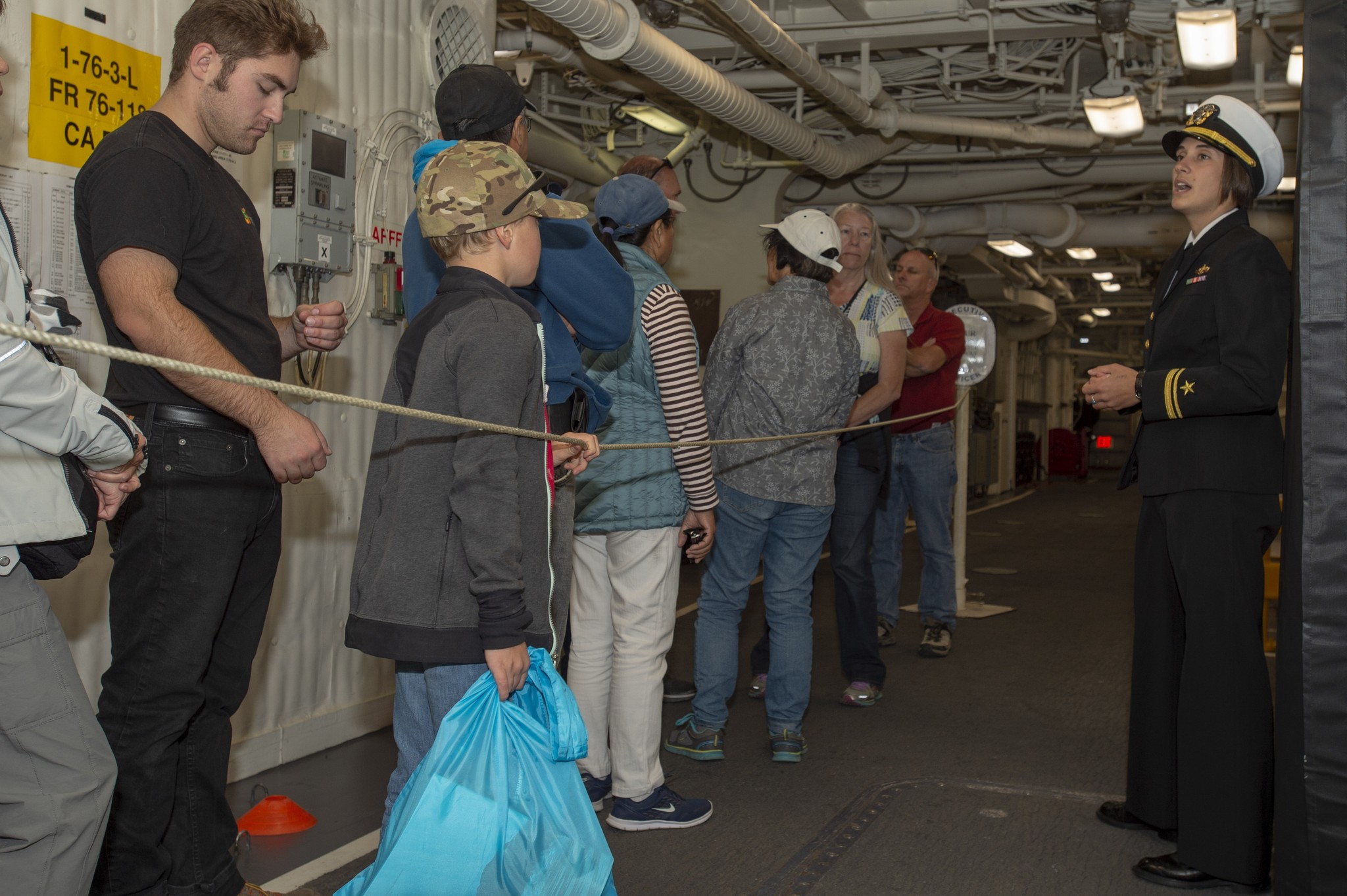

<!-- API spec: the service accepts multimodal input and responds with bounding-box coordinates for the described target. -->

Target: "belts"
[126,404,254,435]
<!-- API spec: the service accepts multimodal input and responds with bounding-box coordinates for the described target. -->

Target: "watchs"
[1135,369,1147,400]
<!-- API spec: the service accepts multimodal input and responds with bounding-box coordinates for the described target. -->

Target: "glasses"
[681,526,707,564]
[916,246,938,267]
[502,170,550,216]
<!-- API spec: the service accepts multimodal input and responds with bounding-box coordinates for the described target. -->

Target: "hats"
[415,138,590,239]
[595,175,688,242]
[758,208,843,273]
[1162,95,1285,199]
[434,63,539,140]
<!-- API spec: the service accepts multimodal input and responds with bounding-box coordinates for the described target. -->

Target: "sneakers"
[877,615,897,645]
[581,772,613,811]
[606,785,714,831]
[664,713,727,760]
[747,674,768,698]
[768,730,809,762]
[842,682,884,706]
[662,674,697,703]
[920,618,952,657]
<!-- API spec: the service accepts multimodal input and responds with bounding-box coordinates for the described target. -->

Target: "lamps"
[984,227,1037,259]
[617,96,698,136]
[1078,60,1145,139]
[1169,1,1240,73]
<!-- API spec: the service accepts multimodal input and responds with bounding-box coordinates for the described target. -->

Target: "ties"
[1177,242,1193,270]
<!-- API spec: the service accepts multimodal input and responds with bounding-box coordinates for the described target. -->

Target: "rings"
[1092,393,1098,404]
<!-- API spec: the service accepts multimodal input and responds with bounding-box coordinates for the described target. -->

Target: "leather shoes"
[1095,800,1178,841]
[1136,852,1241,889]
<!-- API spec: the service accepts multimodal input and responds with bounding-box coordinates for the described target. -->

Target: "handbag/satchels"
[333,646,620,896]
[10,315,100,579]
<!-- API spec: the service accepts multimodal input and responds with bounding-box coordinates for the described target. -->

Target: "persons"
[866,243,967,660]
[70,0,355,896]
[747,202,916,708]
[559,171,720,833]
[403,58,639,680]
[1079,95,1294,896]
[344,140,601,866]
[0,2,149,893]
[663,206,863,768]
[558,154,701,699]
